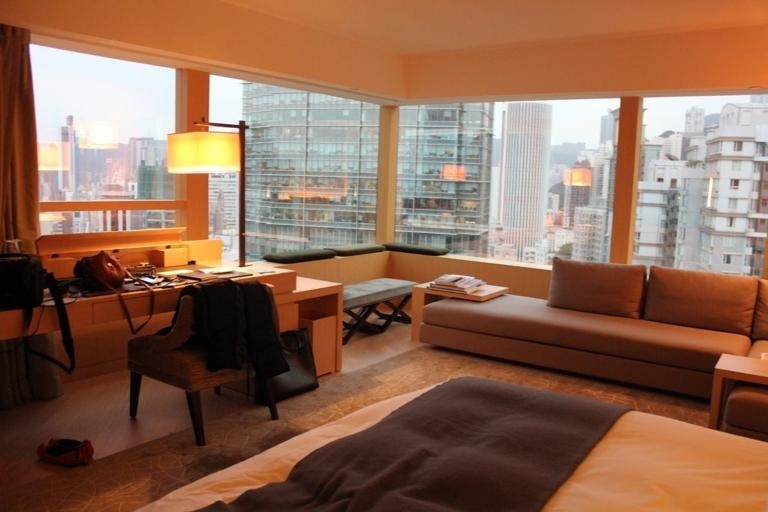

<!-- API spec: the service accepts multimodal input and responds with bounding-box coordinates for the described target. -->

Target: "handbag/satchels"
[73,251,125,290]
[0,253,43,310]
[255,328,318,406]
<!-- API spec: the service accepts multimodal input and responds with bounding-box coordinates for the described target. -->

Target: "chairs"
[126,281,292,446]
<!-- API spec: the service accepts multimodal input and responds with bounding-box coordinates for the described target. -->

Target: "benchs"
[343,277,421,346]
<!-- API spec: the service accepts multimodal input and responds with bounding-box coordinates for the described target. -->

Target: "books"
[156,266,253,287]
[427,274,486,295]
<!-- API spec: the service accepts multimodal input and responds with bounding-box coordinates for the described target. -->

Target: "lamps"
[440,162,469,181]
[563,166,593,190]
[165,118,251,266]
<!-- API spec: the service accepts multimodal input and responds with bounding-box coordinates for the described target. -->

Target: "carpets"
[0,346,709,511]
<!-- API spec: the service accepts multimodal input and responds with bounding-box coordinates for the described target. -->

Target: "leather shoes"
[49,436,93,458]
[37,444,82,467]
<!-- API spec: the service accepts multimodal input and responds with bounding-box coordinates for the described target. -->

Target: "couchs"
[421,256,768,398]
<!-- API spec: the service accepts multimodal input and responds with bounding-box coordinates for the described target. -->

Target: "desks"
[708,352,768,432]
[411,281,510,344]
[0,263,345,379]
